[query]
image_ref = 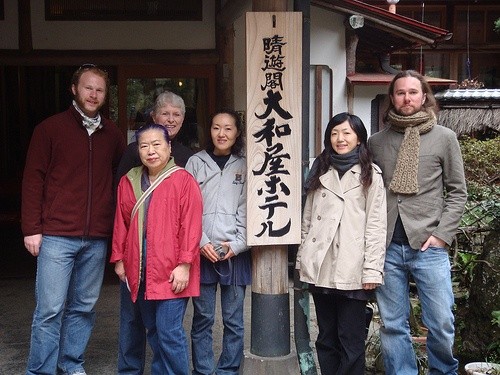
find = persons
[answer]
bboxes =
[365,70,468,375]
[109,123,203,375]
[21,63,120,374]
[112,92,193,375]
[186,110,255,374]
[295,114,387,375]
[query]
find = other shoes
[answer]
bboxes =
[67,371,87,375]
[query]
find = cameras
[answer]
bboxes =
[213,244,229,259]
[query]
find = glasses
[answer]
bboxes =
[74,63,95,74]
